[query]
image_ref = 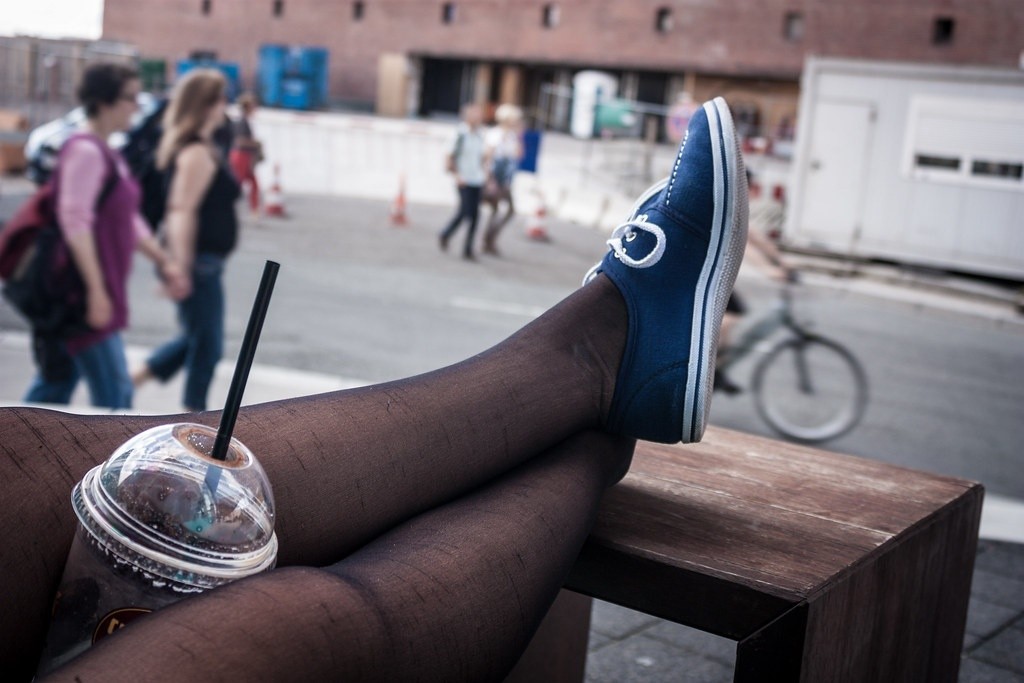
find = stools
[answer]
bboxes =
[505,425,988,681]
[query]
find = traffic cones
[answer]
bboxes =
[260,163,288,220]
[388,183,409,230]
[522,195,551,243]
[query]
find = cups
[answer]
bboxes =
[27,471,278,683]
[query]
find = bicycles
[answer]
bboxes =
[710,260,873,446]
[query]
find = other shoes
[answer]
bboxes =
[437,234,447,248]
[462,250,477,262]
[480,240,499,257]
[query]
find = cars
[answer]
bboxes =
[21,91,161,191]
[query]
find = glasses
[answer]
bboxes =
[121,93,139,103]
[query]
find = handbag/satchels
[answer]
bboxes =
[0,133,119,342]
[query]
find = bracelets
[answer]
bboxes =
[154,251,170,271]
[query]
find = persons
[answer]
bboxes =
[21,59,187,410]
[230,94,262,213]
[130,69,242,413]
[124,99,171,167]
[438,102,541,261]
[715,165,801,394]
[0,94,752,683]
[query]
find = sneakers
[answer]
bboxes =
[580,96,749,444]
